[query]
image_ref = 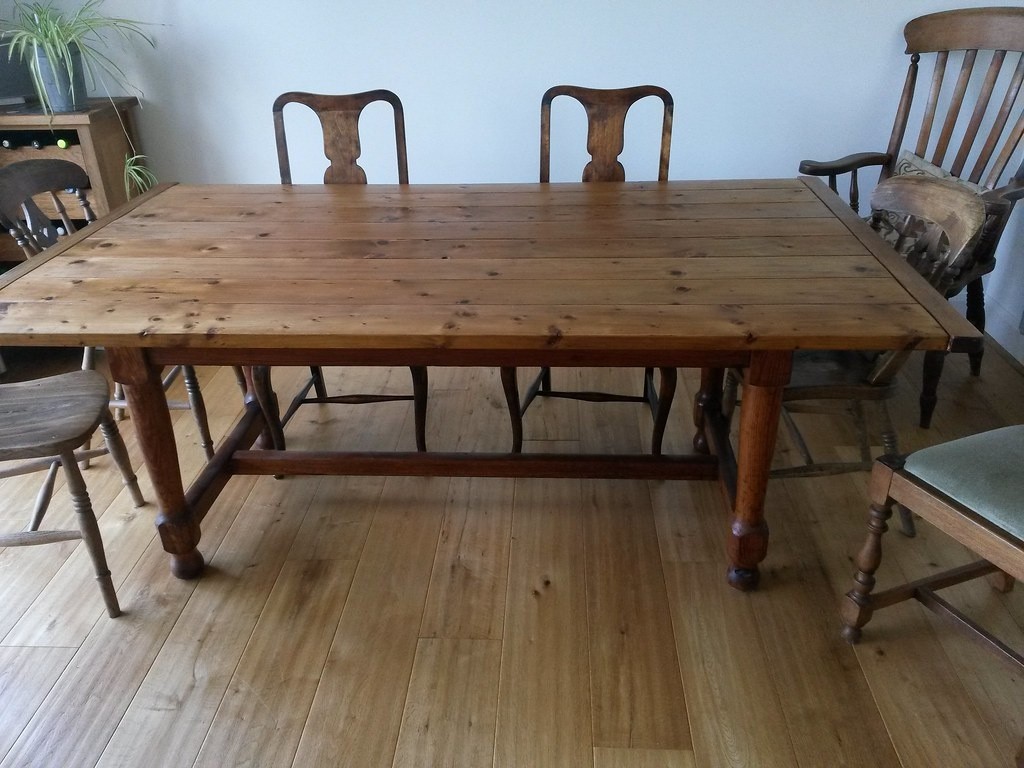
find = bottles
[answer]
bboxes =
[55,226,68,236]
[1,131,79,149]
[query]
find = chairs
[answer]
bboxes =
[796,7,1024,429]
[496,83,681,454]
[0,369,150,619]
[0,158,248,470]
[721,174,989,541]
[246,87,432,481]
[838,419,1024,764]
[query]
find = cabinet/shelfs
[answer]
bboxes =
[0,97,140,360]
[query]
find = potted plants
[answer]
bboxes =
[0,0,173,204]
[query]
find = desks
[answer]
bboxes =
[0,177,987,590]
